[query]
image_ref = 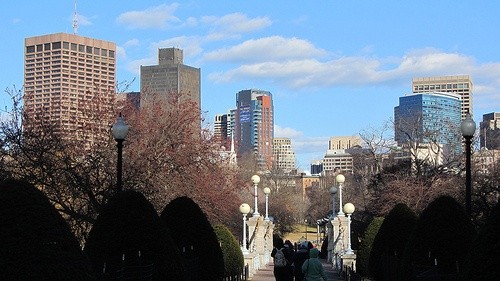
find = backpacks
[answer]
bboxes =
[275,248,289,266]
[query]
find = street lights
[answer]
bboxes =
[329,185,338,218]
[251,175,261,218]
[263,186,271,221]
[239,203,250,252]
[343,202,355,254]
[460,112,476,281]
[335,174,347,217]
[110,111,130,197]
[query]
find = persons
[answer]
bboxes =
[284,239,328,281]
[271,238,294,281]
